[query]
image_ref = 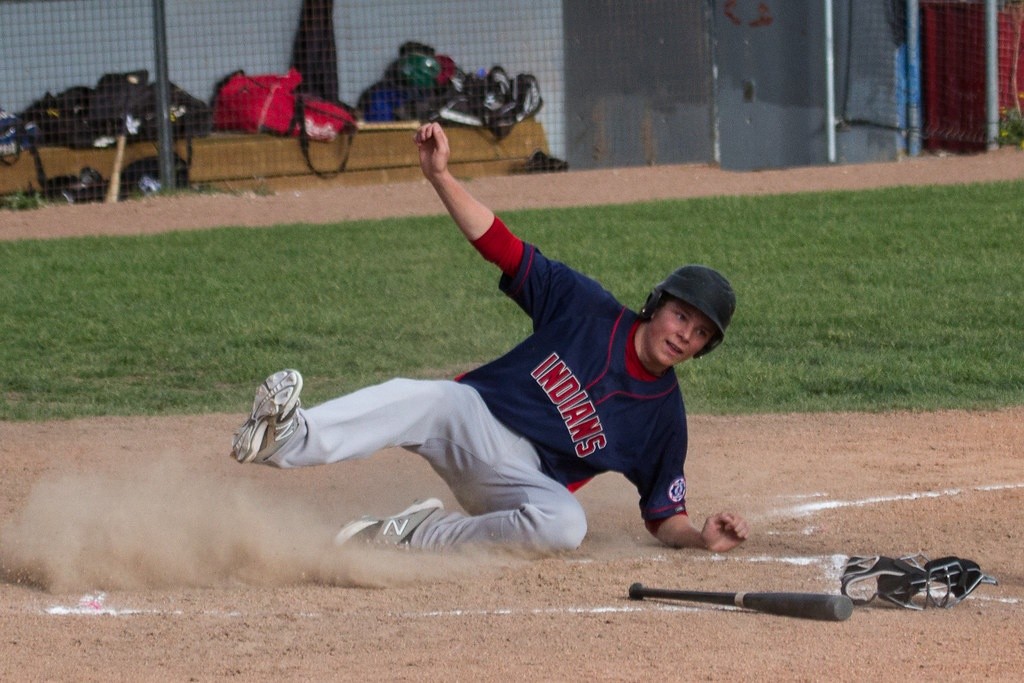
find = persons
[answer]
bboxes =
[231,122,750,558]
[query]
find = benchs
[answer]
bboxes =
[0,116,548,195]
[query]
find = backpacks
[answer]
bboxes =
[360,42,544,136]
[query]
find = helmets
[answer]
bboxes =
[646,266,736,358]
[394,55,445,89]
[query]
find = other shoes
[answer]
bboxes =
[512,151,569,173]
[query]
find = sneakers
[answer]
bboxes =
[332,499,445,549]
[231,367,303,463]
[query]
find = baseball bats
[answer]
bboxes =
[627,580,856,624]
[103,74,139,204]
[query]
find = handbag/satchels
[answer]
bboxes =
[210,71,357,180]
[0,69,208,203]
